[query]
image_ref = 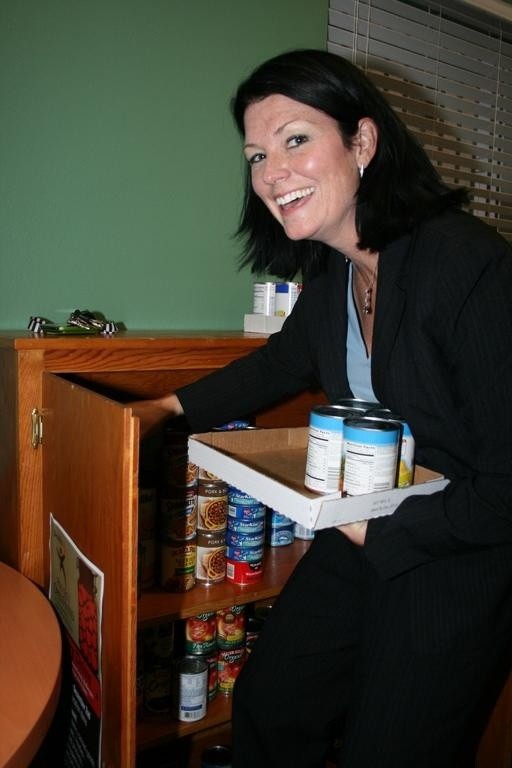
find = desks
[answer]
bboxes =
[0,561,64,768]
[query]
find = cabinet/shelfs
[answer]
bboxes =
[0,330,338,767]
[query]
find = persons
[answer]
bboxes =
[127,51,511,766]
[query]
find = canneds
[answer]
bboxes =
[253,281,303,318]
[136,604,272,723]
[139,418,317,593]
[301,399,416,497]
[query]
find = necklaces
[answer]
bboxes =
[354,260,380,316]
